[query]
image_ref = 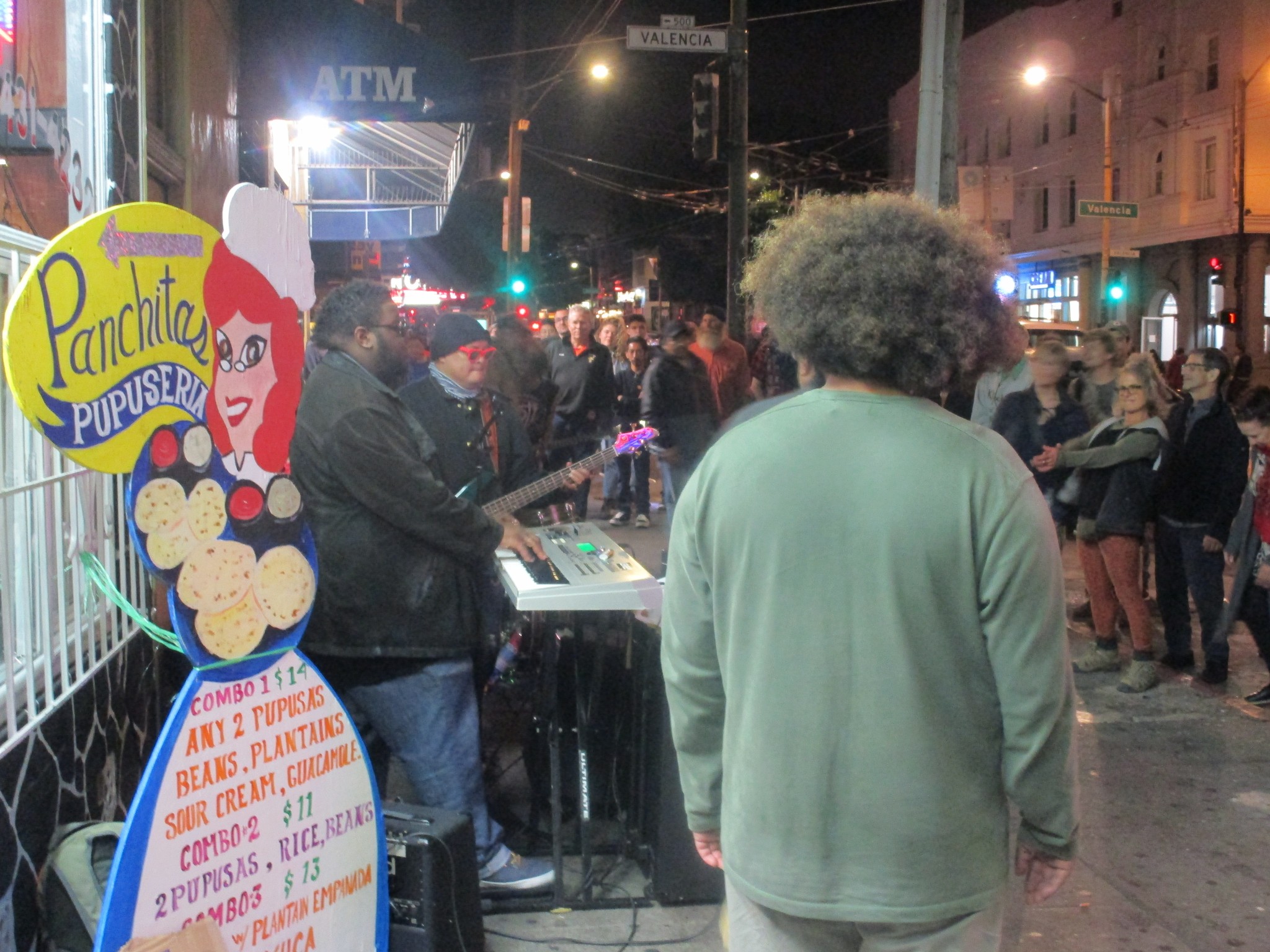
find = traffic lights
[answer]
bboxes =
[1209,257,1224,287]
[1106,283,1126,304]
[1221,309,1237,328]
[513,279,526,296]
[505,64,611,308]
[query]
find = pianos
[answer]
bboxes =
[489,521,665,913]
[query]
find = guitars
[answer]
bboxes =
[483,419,659,522]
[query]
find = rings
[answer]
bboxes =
[1043,459,1045,466]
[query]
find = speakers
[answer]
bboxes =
[634,622,724,904]
[380,800,487,952]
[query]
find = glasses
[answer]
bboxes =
[1181,361,1202,368]
[373,316,407,336]
[459,345,495,362]
[1115,384,1140,394]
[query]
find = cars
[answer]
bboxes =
[1018,318,1087,358]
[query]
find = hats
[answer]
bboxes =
[662,320,687,337]
[702,307,726,322]
[429,313,491,360]
[1105,319,1129,338]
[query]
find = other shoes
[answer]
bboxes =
[1073,638,1120,673]
[609,511,630,525]
[1117,650,1157,692]
[1161,649,1193,667]
[635,515,650,528]
[1067,601,1094,635]
[1194,661,1227,684]
[1244,683,1270,705]
[481,847,555,889]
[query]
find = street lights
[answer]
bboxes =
[1019,57,1117,328]
[570,260,595,313]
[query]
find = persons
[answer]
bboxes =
[660,185,1086,952]
[303,298,1270,852]
[285,278,561,888]
